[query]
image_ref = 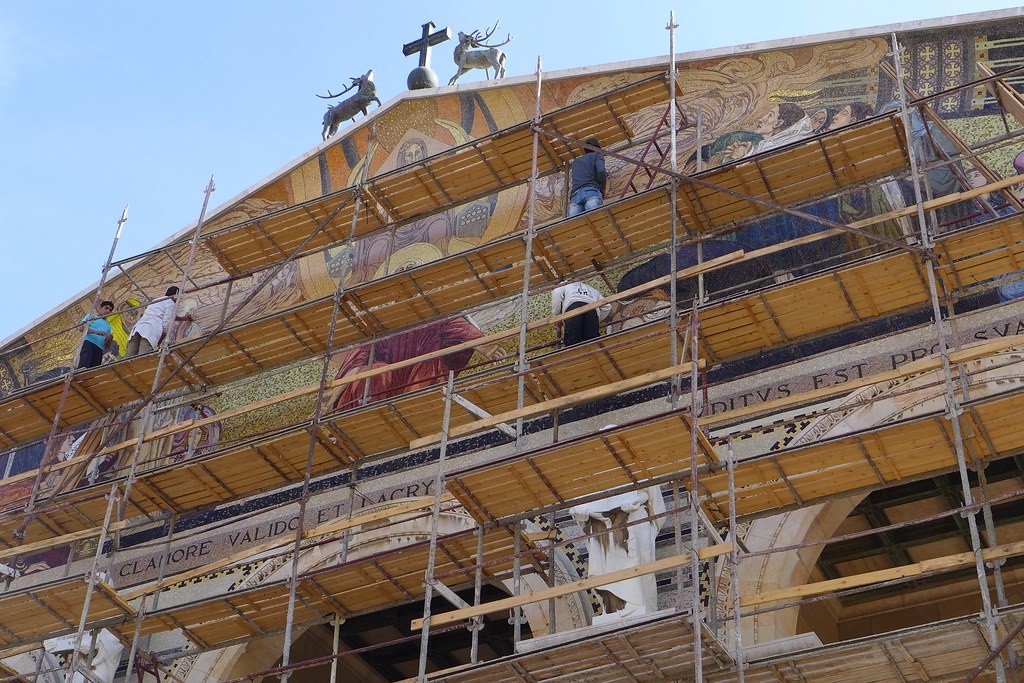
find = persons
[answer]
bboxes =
[567,138,607,219]
[551,282,611,347]
[126,285,193,358]
[78,301,114,369]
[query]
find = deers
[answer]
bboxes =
[315,69,381,141]
[448,20,515,86]
